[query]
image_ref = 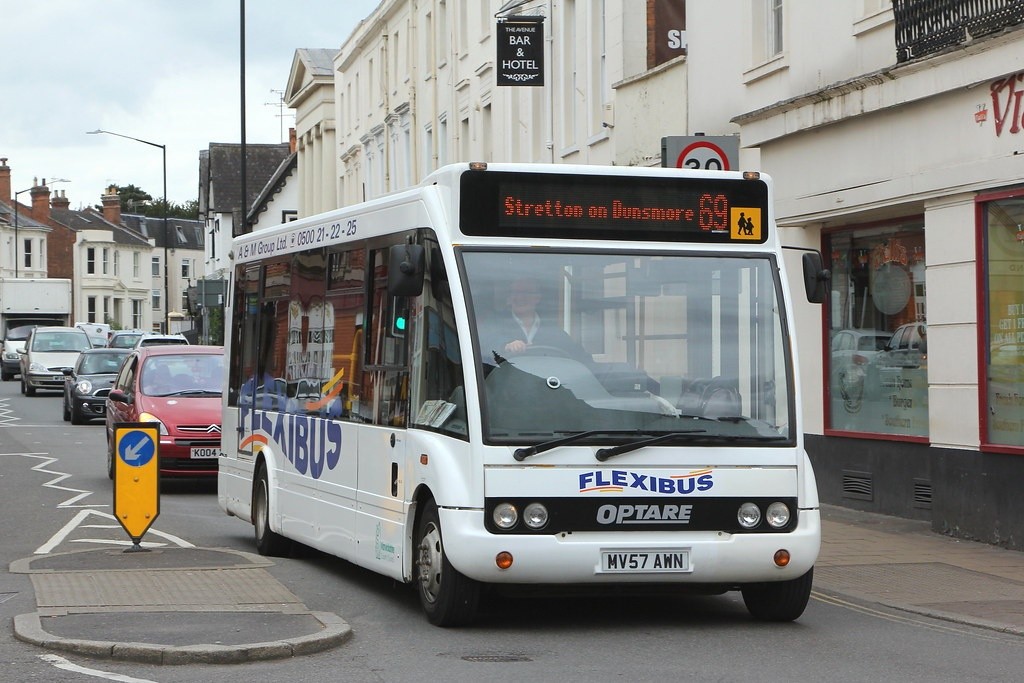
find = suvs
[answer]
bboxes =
[832,330,894,399]
[865,323,927,402]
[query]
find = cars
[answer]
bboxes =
[17,325,95,397]
[237,377,358,416]
[104,346,224,480]
[61,348,137,424]
[988,339,1024,416]
[107,330,156,360]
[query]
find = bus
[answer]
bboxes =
[218,161,832,622]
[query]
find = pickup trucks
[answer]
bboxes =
[132,332,199,377]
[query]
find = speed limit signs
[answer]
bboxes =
[677,145,730,172]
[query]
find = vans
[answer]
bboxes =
[73,322,112,348]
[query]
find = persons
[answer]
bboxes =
[143,364,178,395]
[483,274,594,371]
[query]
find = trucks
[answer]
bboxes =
[0,277,73,383]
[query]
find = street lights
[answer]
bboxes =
[86,129,168,336]
[14,178,72,277]
[197,304,209,345]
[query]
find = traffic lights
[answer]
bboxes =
[387,292,407,339]
